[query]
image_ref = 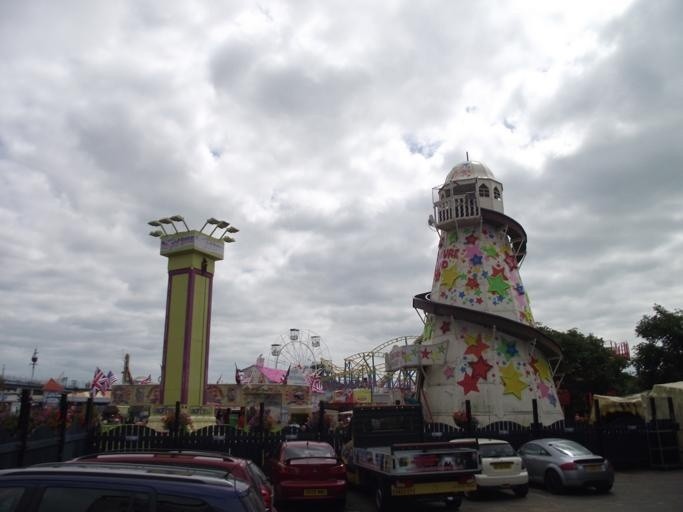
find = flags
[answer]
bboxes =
[90,367,118,397]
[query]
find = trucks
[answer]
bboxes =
[341,399,482,511]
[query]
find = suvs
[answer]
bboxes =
[0,461,265,512]
[65,449,278,512]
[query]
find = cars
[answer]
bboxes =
[276,441,347,501]
[452,424,615,500]
